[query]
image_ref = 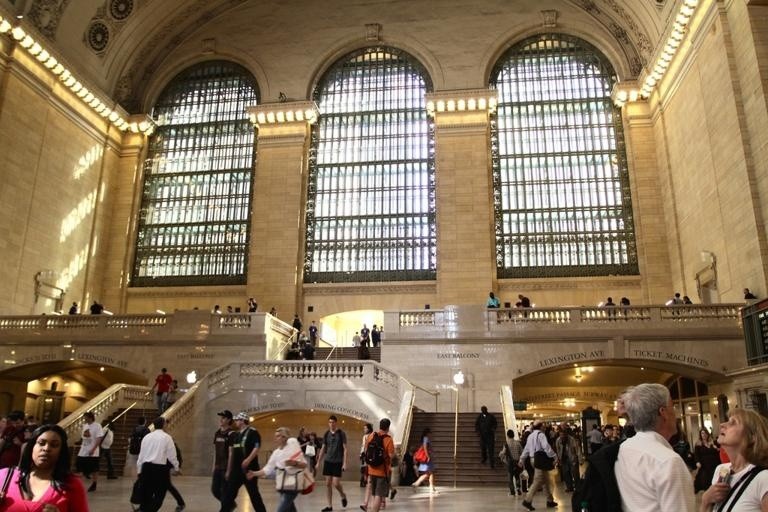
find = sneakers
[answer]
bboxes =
[321,488,397,512]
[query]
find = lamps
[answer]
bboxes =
[1,12,159,139]
[610,0,701,110]
[247,107,321,127]
[421,94,498,117]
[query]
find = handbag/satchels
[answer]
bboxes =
[302,467,316,494]
[506,447,524,475]
[274,465,304,492]
[534,449,555,470]
[415,448,427,463]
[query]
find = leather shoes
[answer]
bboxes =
[522,500,535,511]
[546,501,558,508]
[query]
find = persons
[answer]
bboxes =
[220,412,269,512]
[671,292,682,315]
[604,296,617,321]
[1,409,113,512]
[353,323,381,360]
[225,306,232,313]
[165,381,181,402]
[246,297,257,311]
[683,295,692,304]
[486,292,501,310]
[164,445,185,512]
[209,410,237,512]
[213,303,222,313]
[133,417,152,462]
[298,412,399,511]
[411,427,437,493]
[620,296,631,320]
[742,287,756,300]
[130,415,182,512]
[89,300,102,314]
[515,302,522,308]
[285,314,317,361]
[269,307,276,317]
[149,368,173,414]
[500,384,768,512]
[516,294,529,320]
[247,427,308,512]
[475,404,497,469]
[69,301,81,314]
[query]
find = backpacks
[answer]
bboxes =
[129,426,146,454]
[365,432,391,467]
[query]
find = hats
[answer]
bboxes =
[233,412,249,420]
[217,410,233,419]
